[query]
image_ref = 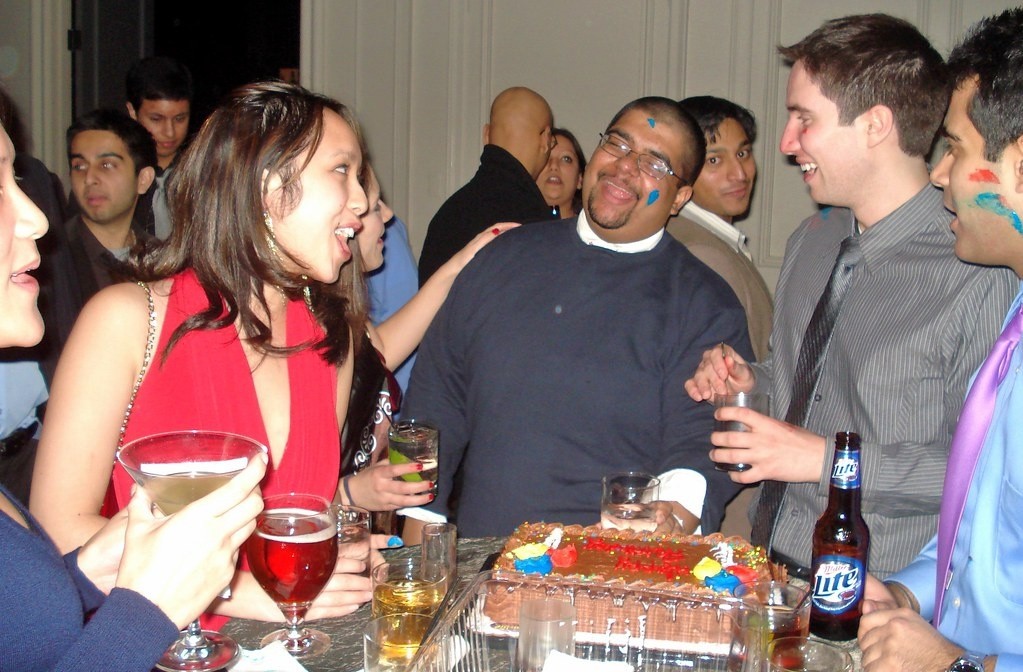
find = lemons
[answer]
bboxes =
[388,448,422,482]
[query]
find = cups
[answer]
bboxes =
[763,637,852,672]
[325,505,372,608]
[729,581,813,672]
[371,558,448,646]
[420,522,457,588]
[363,613,437,672]
[713,392,769,474]
[516,599,577,672]
[601,470,661,534]
[388,421,438,498]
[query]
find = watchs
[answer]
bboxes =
[945,650,987,672]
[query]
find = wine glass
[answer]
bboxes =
[116,430,267,672]
[246,493,338,660]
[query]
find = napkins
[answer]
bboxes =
[226,640,308,672]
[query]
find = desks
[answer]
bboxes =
[218,536,858,672]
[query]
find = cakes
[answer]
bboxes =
[483,521,789,645]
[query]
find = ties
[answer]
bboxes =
[932,305,1023,630]
[750,236,862,557]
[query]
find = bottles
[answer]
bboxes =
[548,204,561,220]
[808,432,871,641]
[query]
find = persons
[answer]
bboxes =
[534,127,587,219]
[685,12,1020,592]
[397,94,756,544]
[798,3,1023,672]
[416,88,563,289]
[0,52,519,672]
[665,95,776,365]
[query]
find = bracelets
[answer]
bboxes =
[343,474,357,507]
[888,581,915,611]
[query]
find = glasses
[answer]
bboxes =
[599,133,690,186]
